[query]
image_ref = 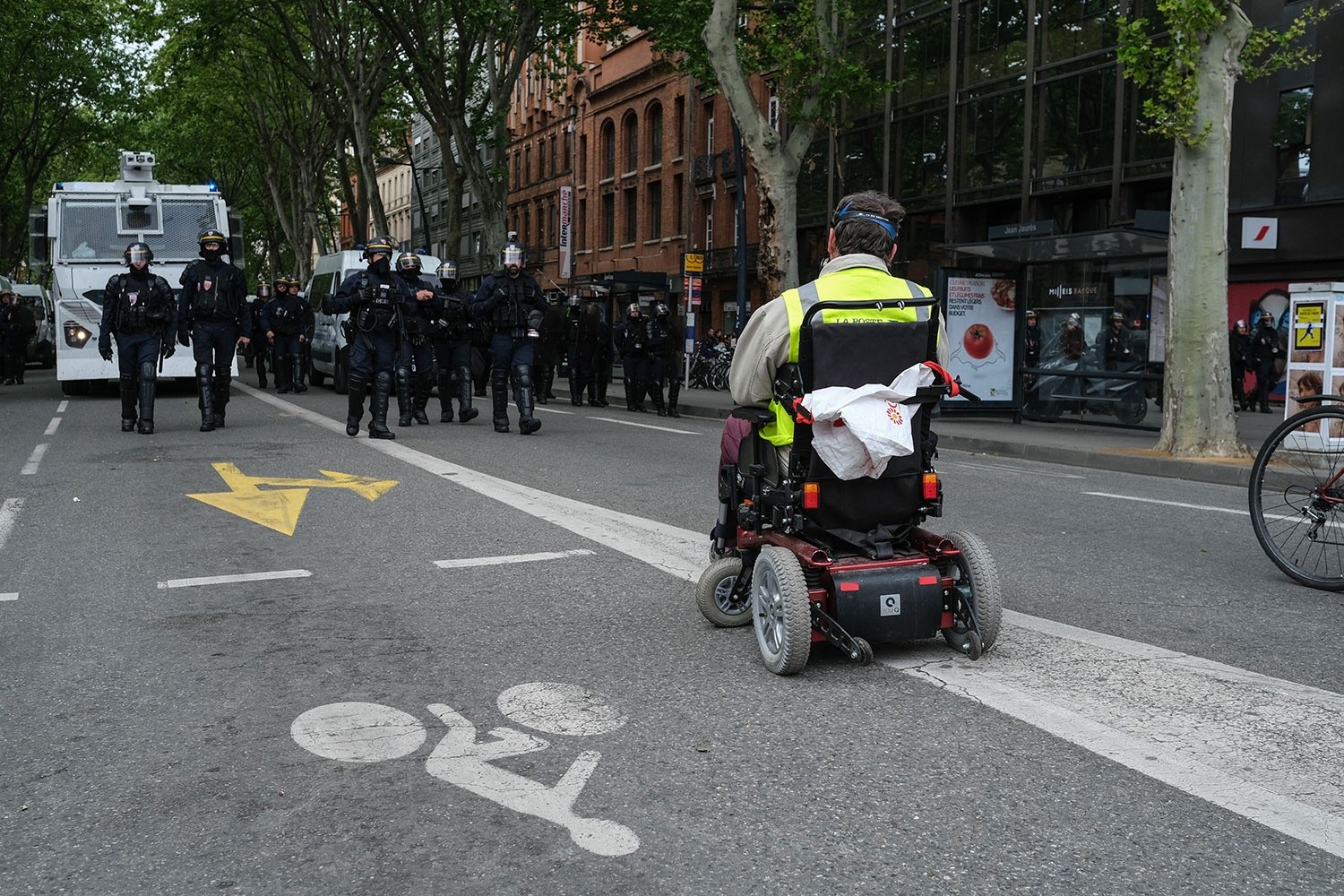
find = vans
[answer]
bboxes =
[0,274,57,371]
[302,244,442,397]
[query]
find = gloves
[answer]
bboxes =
[177,330,190,347]
[98,343,114,361]
[525,287,537,300]
[360,287,372,301]
[495,284,509,298]
[162,341,176,358]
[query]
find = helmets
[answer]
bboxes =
[569,293,582,306]
[395,251,422,274]
[626,303,642,316]
[1234,319,1248,328]
[257,281,270,296]
[361,236,392,261]
[11,293,24,306]
[273,274,302,288]
[1,288,12,296]
[1111,312,1124,320]
[654,303,672,316]
[124,242,154,268]
[500,241,526,266]
[1260,312,1274,318]
[197,227,225,245]
[435,260,460,282]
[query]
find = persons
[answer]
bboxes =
[1023,310,1041,367]
[1244,312,1288,414]
[0,289,37,385]
[98,242,177,435]
[1094,312,1131,372]
[329,237,413,440]
[473,289,739,418]
[1228,319,1254,411]
[253,274,315,394]
[1058,313,1090,358]
[473,240,547,435]
[393,252,444,427]
[717,189,951,499]
[177,228,251,432]
[1292,372,1322,433]
[434,261,479,423]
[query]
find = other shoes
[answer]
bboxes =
[458,407,479,423]
[398,415,412,427]
[259,378,308,394]
[411,408,429,425]
[441,409,454,422]
[136,418,154,435]
[1246,395,1256,412]
[492,416,510,433]
[1260,403,1273,414]
[121,418,136,432]
[518,418,542,435]
[657,406,666,417]
[346,414,363,436]
[1238,394,1247,411]
[200,414,226,432]
[367,420,396,440]
[572,394,648,412]
[5,377,24,386]
[667,408,680,418]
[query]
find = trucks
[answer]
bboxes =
[45,150,247,396]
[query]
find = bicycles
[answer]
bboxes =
[1247,393,1344,595]
[679,339,733,394]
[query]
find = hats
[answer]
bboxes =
[1026,311,1036,317]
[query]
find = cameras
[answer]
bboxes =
[508,231,518,240]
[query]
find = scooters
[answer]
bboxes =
[695,294,1005,677]
[1021,326,1153,424]
